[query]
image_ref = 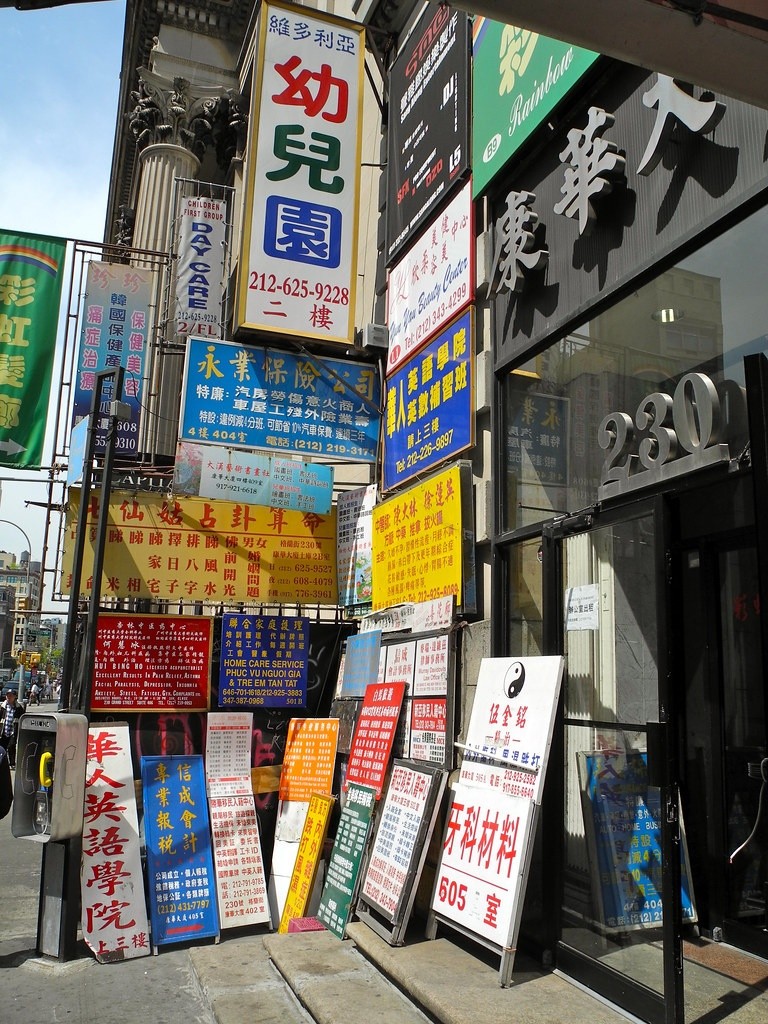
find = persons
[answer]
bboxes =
[0,705,13,820]
[56,683,62,709]
[0,689,25,770]
[28,681,53,706]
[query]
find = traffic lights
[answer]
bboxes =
[19,652,26,663]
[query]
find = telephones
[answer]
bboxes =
[39,751,53,789]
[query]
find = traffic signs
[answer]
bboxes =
[14,633,36,643]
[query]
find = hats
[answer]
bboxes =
[6,689,16,696]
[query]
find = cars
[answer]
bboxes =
[1,680,20,701]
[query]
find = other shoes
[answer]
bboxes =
[10,765,16,770]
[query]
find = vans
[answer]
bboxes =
[0,668,52,699]
